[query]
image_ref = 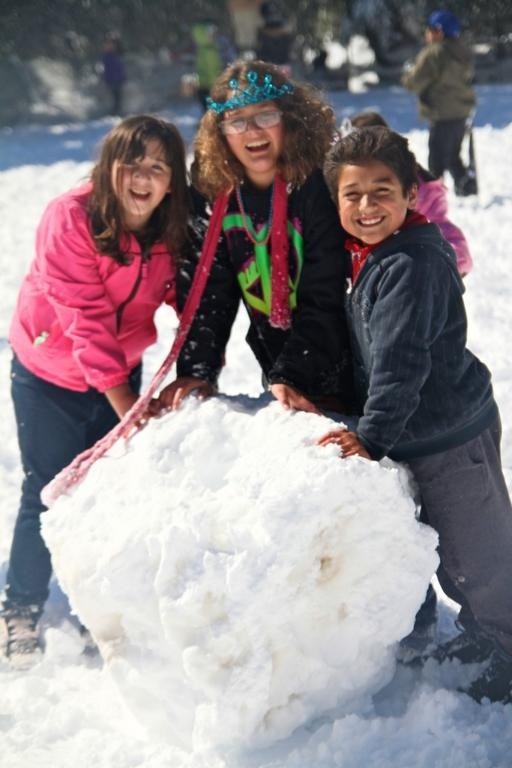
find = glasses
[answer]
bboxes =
[218,110,284,135]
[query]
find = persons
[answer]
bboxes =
[95,30,126,119]
[312,122,511,710]
[2,110,193,669]
[186,26,222,112]
[336,111,476,282]
[400,9,481,197]
[158,52,440,664]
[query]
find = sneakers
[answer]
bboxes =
[399,584,438,668]
[412,618,495,671]
[1,604,45,668]
[457,660,512,706]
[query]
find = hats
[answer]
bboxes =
[423,10,461,38]
